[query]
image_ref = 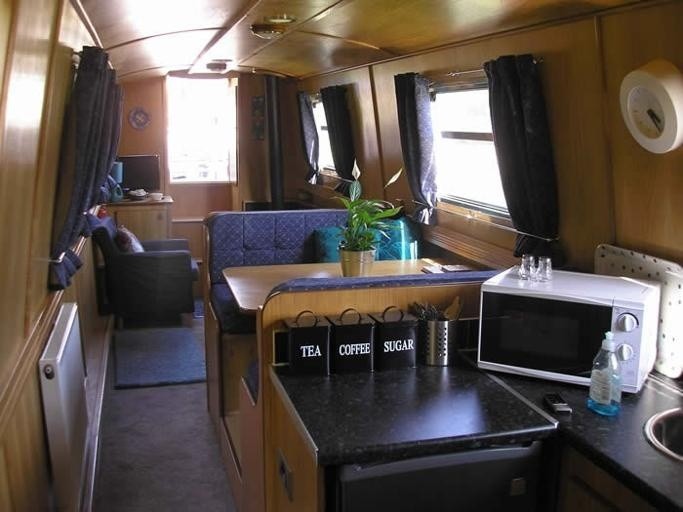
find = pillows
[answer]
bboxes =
[374,214,432,259]
[311,226,381,263]
[115,222,145,253]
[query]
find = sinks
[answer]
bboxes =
[644,407,683,461]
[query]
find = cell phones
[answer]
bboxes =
[542,392,573,414]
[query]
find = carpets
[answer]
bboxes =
[189,293,205,318]
[113,327,206,388]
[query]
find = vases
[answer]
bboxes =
[337,245,377,277]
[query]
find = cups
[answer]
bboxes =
[519,254,535,280]
[536,257,553,282]
[149,193,162,201]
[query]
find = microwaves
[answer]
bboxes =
[477,264,662,393]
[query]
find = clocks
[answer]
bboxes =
[618,58,682,155]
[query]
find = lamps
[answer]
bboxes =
[112,162,123,184]
[250,22,292,42]
[206,63,227,73]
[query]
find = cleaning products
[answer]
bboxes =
[587,331,623,416]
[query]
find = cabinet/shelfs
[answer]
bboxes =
[103,194,175,241]
[556,442,654,512]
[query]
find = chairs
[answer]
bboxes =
[93,216,200,314]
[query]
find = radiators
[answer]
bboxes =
[38,300,90,512]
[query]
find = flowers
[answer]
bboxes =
[329,158,403,252]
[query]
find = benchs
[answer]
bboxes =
[199,210,423,421]
[242,269,512,512]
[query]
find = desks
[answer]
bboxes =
[222,258,471,311]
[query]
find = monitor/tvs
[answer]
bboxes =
[116,155,160,191]
